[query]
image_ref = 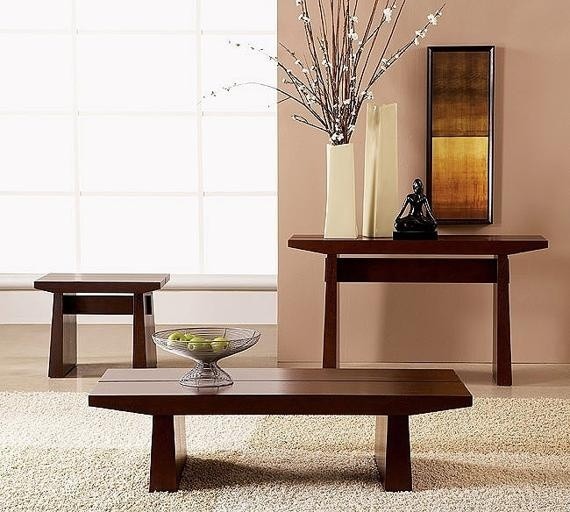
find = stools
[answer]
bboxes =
[33,272,170,378]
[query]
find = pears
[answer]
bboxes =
[166,332,232,354]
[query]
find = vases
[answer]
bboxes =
[361,100,399,238]
[324,143,358,239]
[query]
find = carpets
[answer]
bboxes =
[0,390,570,511]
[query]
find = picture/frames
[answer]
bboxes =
[427,44,495,226]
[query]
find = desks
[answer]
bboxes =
[88,368,473,492]
[286,233,550,386]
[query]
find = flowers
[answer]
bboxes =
[199,0,448,146]
